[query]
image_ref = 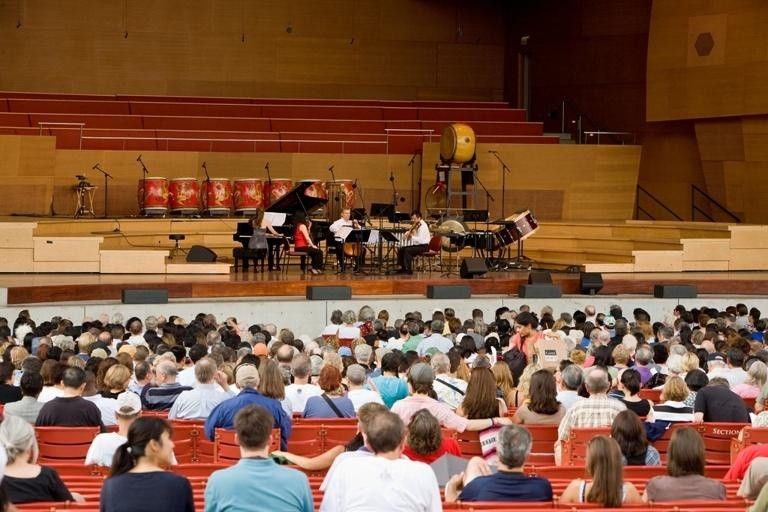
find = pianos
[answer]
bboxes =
[233,183,330,271]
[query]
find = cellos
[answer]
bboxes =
[344,210,364,258]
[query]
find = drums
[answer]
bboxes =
[497,208,539,247]
[439,124,475,165]
[137,176,355,215]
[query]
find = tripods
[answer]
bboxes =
[94,175,120,219]
[440,236,461,278]
[127,170,165,218]
[194,182,222,218]
[334,213,401,276]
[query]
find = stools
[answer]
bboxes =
[168,234,185,260]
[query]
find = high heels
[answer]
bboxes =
[311,269,324,275]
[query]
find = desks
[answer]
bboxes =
[71,186,99,217]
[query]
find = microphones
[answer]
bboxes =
[265,162,269,169]
[136,155,141,161]
[329,165,334,171]
[92,163,99,169]
[202,161,205,168]
[488,151,497,153]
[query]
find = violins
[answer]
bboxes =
[407,222,422,240]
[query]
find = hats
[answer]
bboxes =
[604,316,616,326]
[410,363,435,383]
[236,365,259,388]
[116,391,141,416]
[338,346,353,356]
[707,353,727,363]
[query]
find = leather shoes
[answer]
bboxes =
[396,270,413,275]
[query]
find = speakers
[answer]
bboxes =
[461,258,488,278]
[579,272,603,295]
[186,245,217,262]
[528,272,553,285]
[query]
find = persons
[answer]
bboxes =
[246,204,284,271]
[203,404,315,511]
[721,441,767,512]
[445,423,554,503]
[99,415,196,511]
[642,426,727,500]
[327,207,365,272]
[1,301,766,471]
[0,415,76,502]
[292,210,325,276]
[392,209,432,275]
[557,434,643,504]
[321,411,442,511]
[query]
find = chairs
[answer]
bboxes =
[282,235,443,274]
[0,335,768,512]
